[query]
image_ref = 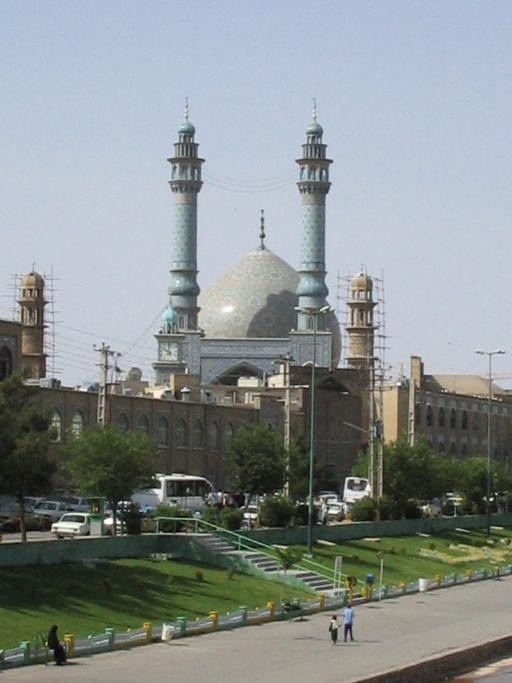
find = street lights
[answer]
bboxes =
[293,306,334,554]
[476,349,505,536]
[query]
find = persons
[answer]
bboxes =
[321,499,329,524]
[329,615,339,643]
[186,486,245,509]
[344,604,353,642]
[48,625,66,666]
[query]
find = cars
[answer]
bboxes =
[239,505,260,527]
[1,495,132,537]
[315,489,345,521]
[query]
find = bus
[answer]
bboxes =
[134,473,217,515]
[342,476,372,519]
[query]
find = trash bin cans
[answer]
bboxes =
[140,512,154,533]
[161,624,174,641]
[418,578,430,592]
